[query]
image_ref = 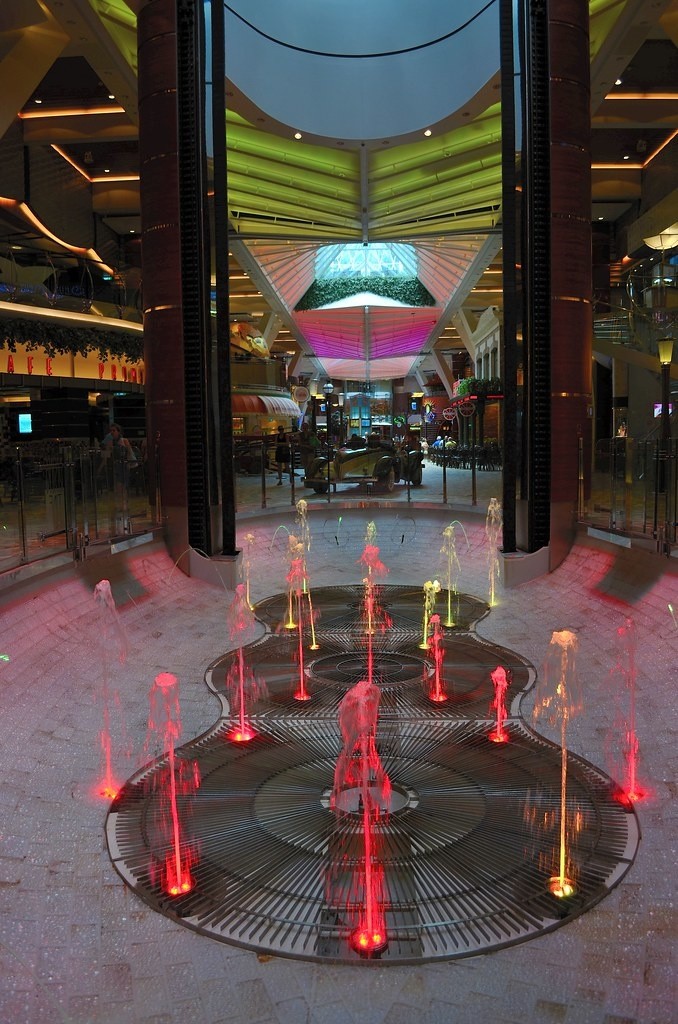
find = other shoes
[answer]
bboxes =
[277,482,282,485]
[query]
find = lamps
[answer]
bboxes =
[657,335,676,363]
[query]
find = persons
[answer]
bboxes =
[107,424,138,473]
[421,436,456,454]
[274,423,328,486]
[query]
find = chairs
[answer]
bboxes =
[428,446,501,471]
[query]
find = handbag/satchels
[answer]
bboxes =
[96,453,114,483]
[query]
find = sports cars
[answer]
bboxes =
[303,441,427,495]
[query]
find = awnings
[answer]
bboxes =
[232,394,301,417]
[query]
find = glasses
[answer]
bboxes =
[279,429,284,430]
